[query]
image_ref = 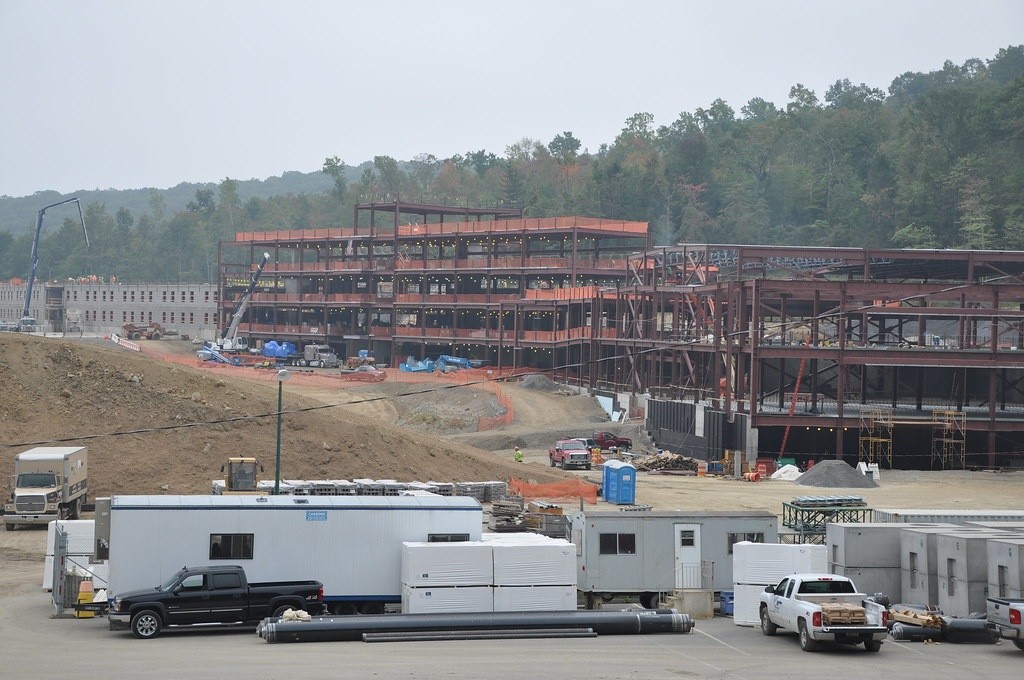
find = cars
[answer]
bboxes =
[1,321,21,333]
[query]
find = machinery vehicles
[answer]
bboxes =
[18,195,91,332]
[217,453,266,496]
[212,253,270,352]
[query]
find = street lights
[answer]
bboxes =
[273,363,288,493]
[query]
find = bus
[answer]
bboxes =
[122,321,163,341]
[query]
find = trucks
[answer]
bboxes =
[4,444,87,530]
[285,345,338,369]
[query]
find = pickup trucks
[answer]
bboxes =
[338,365,386,383]
[586,432,632,454]
[759,575,889,656]
[984,596,1023,651]
[111,563,325,639]
[548,437,591,471]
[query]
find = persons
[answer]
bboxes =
[34,274,117,285]
[514,446,523,462]
